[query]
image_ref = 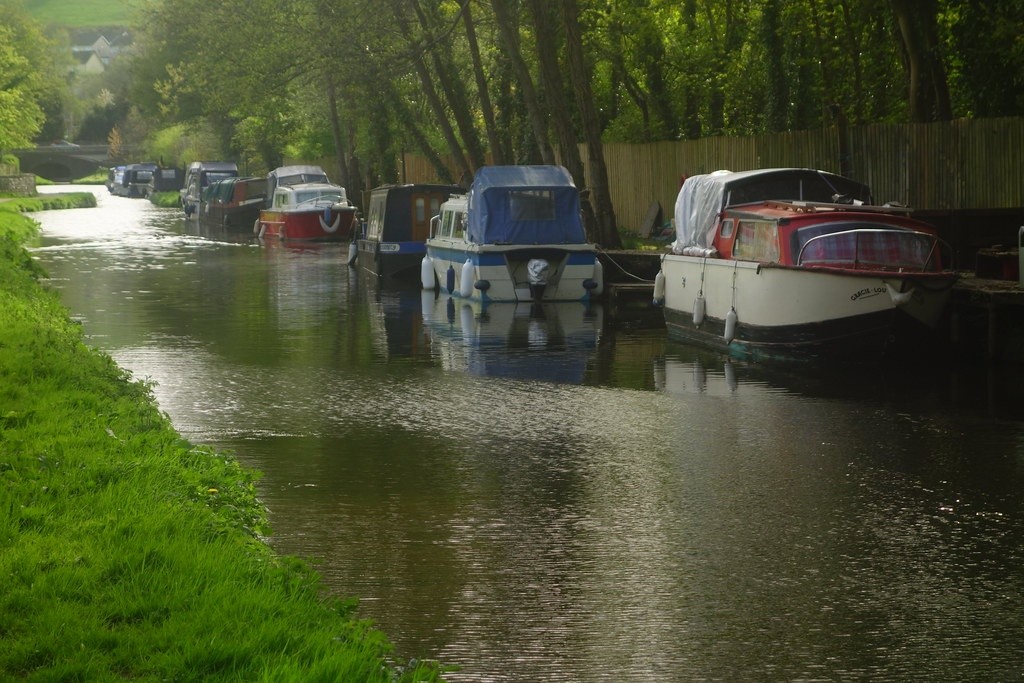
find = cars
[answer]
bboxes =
[49,140,80,152]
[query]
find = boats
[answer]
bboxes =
[653,167,959,376]
[108,162,186,200]
[347,184,467,279]
[420,288,605,359]
[420,165,604,303]
[253,183,358,242]
[266,164,332,208]
[180,162,266,225]
